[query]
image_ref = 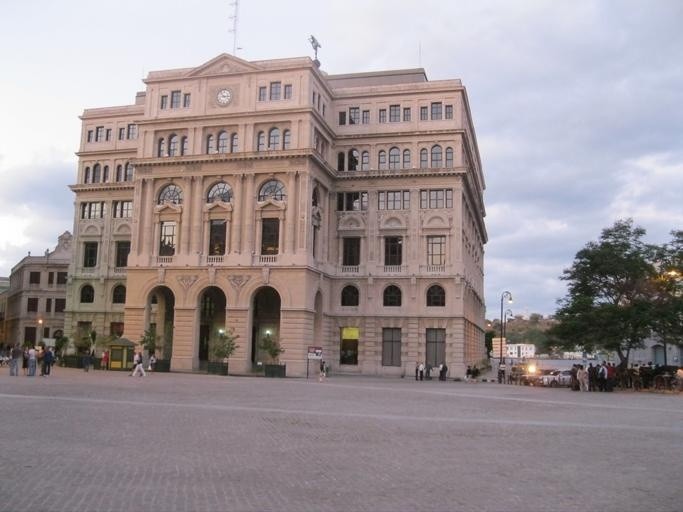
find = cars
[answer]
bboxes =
[511,365,572,388]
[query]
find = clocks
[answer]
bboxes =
[214,87,232,105]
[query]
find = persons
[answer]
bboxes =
[0,342,157,377]
[319,359,327,377]
[414,357,679,392]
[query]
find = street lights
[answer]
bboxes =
[658,258,678,373]
[497,291,513,383]
[504,309,513,337]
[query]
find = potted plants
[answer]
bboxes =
[140,324,170,372]
[55,325,103,369]
[205,326,239,376]
[258,333,288,379]
